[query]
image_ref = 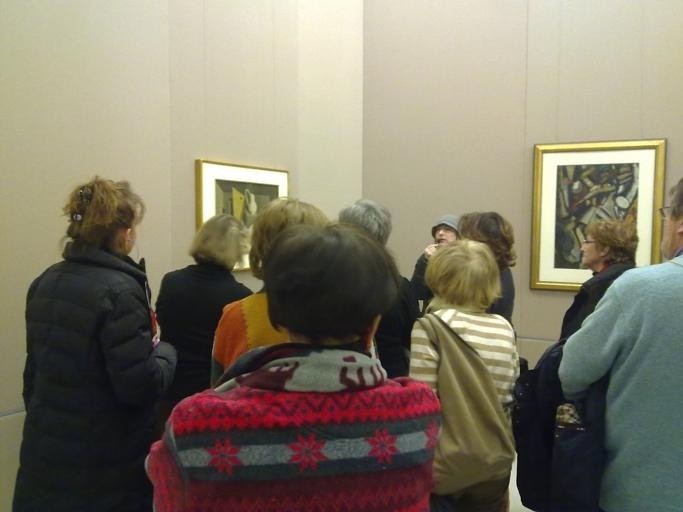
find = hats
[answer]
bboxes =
[432,214,459,236]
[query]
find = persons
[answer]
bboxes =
[154,195,522,511]
[559,213,638,340]
[12,175,176,512]
[556,177,683,512]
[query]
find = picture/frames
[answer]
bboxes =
[195,158,289,272]
[530,137,666,290]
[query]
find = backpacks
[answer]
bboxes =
[512,338,577,511]
